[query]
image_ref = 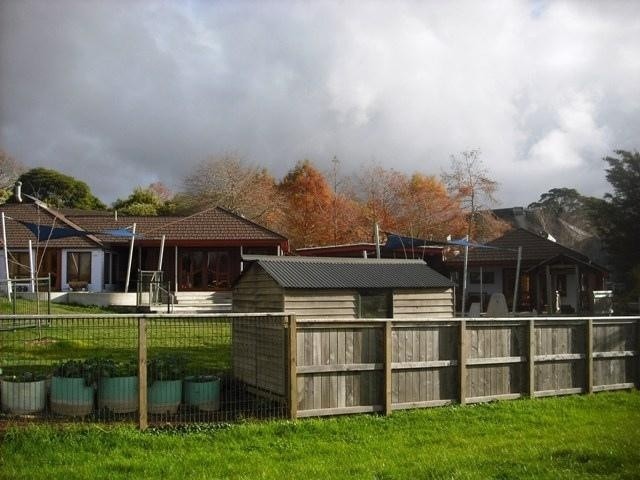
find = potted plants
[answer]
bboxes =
[95,354,139,414]
[50,360,95,416]
[145,360,182,414]
[183,376,221,412]
[1,370,47,416]
